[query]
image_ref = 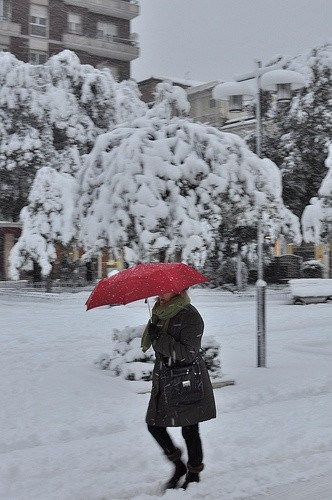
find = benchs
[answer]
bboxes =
[287,279,332,306]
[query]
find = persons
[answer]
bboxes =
[140,287,217,489]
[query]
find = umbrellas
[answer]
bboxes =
[84,262,208,324]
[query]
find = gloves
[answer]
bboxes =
[147,322,160,340]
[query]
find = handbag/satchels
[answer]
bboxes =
[163,363,204,407]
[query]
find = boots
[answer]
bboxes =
[165,450,187,488]
[180,463,205,489]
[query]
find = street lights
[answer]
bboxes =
[212,70,309,369]
